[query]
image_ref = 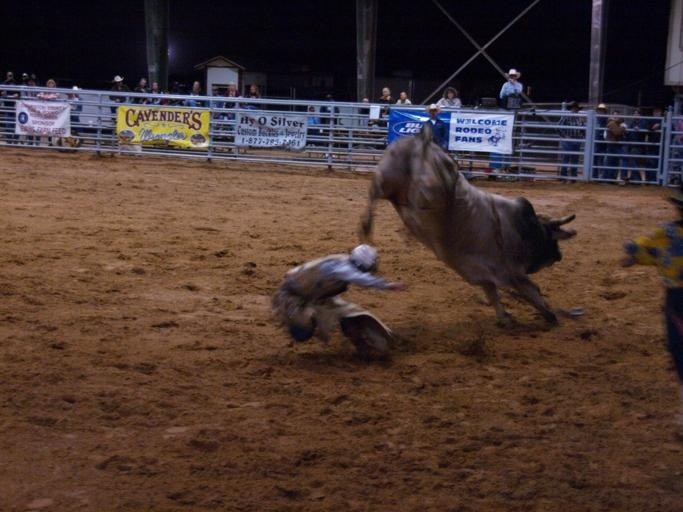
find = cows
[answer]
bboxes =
[359,122,576,332]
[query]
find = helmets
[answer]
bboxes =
[350,243,378,271]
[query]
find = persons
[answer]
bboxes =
[556,101,672,183]
[271,241,408,368]
[2,72,85,147]
[306,93,339,147]
[617,188,683,387]
[364,69,523,177]
[114,75,261,108]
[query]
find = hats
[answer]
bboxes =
[426,103,440,114]
[503,68,521,80]
[444,86,458,98]
[566,100,583,110]
[596,102,608,112]
[111,76,125,83]
